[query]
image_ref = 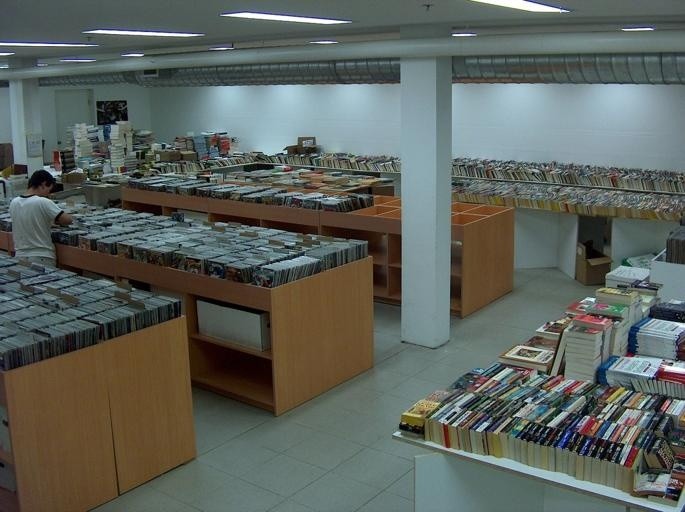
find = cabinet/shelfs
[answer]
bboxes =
[0,201,375,512]
[121,184,514,320]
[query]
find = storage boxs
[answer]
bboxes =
[577,240,611,285]
